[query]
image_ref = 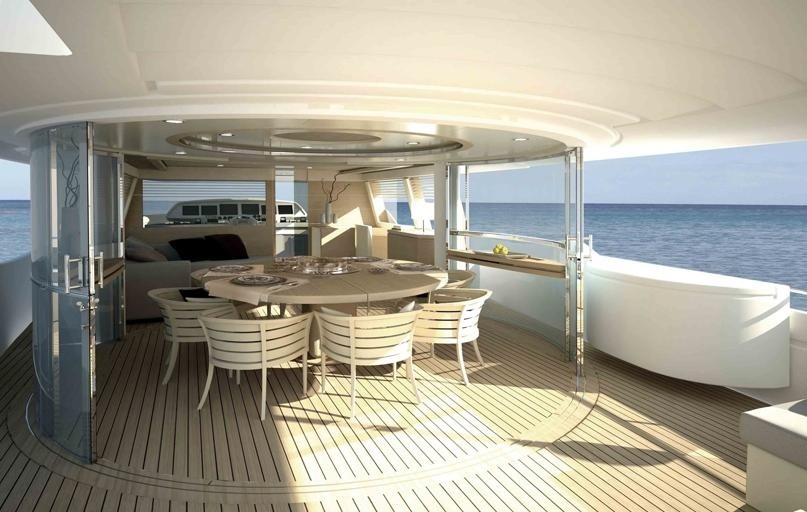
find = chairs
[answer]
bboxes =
[446,268,478,288]
[197,304,314,422]
[149,286,240,386]
[305,301,424,420]
[406,287,493,386]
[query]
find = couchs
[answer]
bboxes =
[125,238,272,322]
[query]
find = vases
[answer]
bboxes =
[325,202,332,224]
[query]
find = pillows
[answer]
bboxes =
[125,233,249,262]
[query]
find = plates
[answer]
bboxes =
[341,256,438,271]
[208,264,289,286]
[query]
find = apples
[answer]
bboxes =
[493,244,508,254]
